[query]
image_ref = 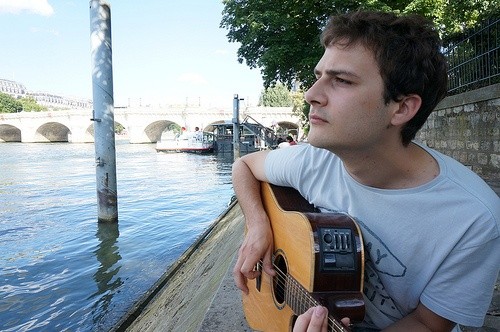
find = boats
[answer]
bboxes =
[155,125,217,154]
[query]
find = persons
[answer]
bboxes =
[278,136,297,149]
[231,6,499,332]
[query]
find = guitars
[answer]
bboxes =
[240,181,368,332]
